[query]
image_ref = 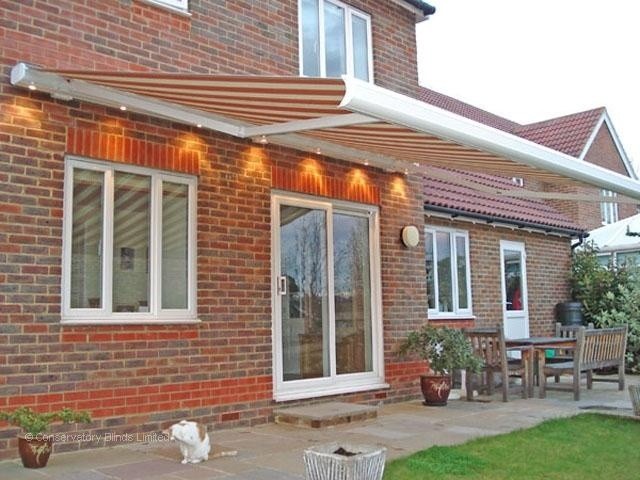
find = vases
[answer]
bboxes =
[301,439,388,480]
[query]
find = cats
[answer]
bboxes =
[162,420,238,464]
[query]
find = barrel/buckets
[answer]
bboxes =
[556,302,585,337]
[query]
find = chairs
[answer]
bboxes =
[461,324,530,402]
[556,320,595,383]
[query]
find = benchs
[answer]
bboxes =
[539,323,629,401]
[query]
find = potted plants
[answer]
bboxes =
[0,405,94,468]
[392,322,484,406]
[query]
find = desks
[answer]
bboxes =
[482,337,577,398]
